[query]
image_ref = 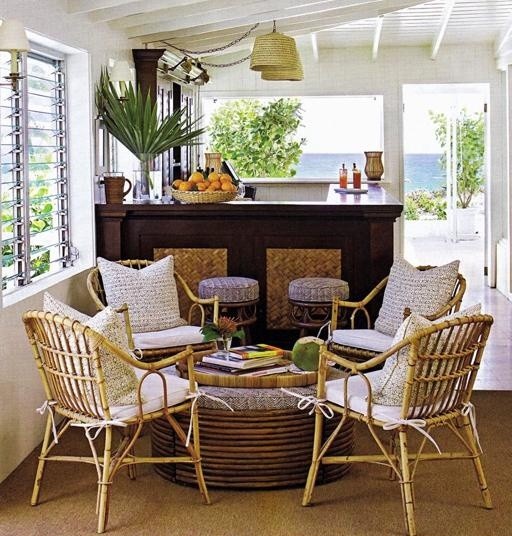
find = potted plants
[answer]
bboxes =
[93,63,208,205]
[426,105,488,244]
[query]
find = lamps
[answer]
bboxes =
[246,18,304,87]
[1,17,32,92]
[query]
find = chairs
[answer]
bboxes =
[327,264,467,437]
[22,309,212,536]
[85,259,220,364]
[301,314,494,535]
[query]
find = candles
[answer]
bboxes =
[351,162,361,189]
[339,163,348,188]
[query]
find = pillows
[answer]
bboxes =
[43,291,148,407]
[97,255,188,332]
[366,303,481,407]
[374,253,460,339]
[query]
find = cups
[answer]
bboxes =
[353,170,361,189]
[364,151,384,181]
[205,152,222,172]
[103,176,132,204]
[339,168,348,188]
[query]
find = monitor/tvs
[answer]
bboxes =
[222,160,240,181]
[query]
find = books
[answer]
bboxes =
[192,341,291,377]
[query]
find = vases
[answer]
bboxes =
[204,152,223,174]
[361,151,385,182]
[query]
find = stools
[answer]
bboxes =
[286,276,349,346]
[198,276,261,347]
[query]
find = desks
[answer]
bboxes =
[146,365,354,490]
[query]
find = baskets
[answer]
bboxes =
[170,189,237,206]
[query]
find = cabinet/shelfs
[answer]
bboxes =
[129,43,211,197]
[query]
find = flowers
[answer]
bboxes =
[201,315,243,351]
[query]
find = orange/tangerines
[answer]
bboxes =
[172,171,238,192]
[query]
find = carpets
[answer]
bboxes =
[1,390,511,536]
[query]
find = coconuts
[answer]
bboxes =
[291,336,326,371]
